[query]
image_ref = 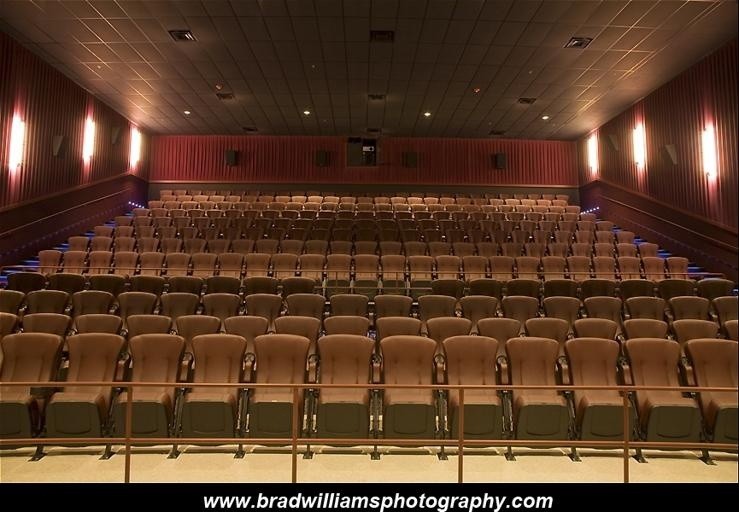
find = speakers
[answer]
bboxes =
[52,135,69,158]
[315,149,327,167]
[225,149,237,166]
[404,151,418,168]
[110,126,123,145]
[496,152,507,169]
[605,134,619,152]
[659,143,678,166]
